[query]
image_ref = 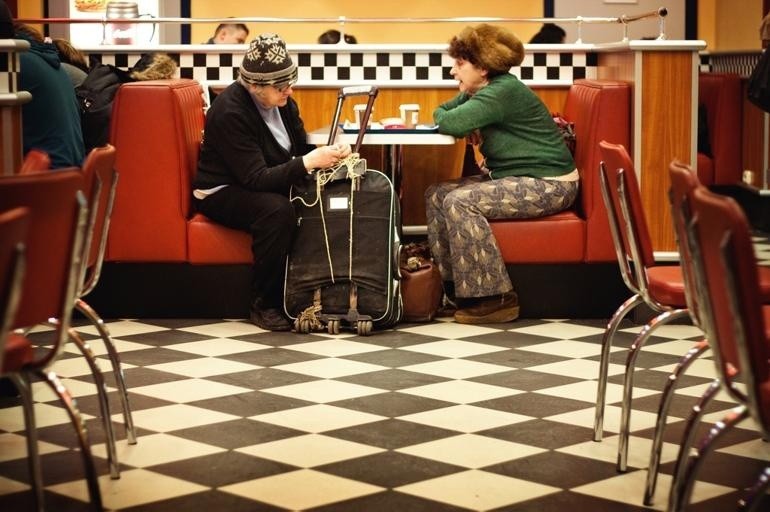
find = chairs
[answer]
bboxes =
[595,141,692,472]
[1,206,47,511]
[23,141,137,483]
[675,182,762,505]
[3,171,112,512]
[646,159,763,505]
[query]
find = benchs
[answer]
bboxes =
[108,78,254,314]
[471,78,629,320]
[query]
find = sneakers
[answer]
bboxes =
[455,292,524,324]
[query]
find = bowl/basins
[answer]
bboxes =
[379,116,403,125]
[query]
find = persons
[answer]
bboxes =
[203,17,248,46]
[192,34,352,331]
[528,23,566,44]
[0,0,86,171]
[11,20,89,93]
[424,25,581,325]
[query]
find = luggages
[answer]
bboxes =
[283,84,404,335]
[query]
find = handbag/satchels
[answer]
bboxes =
[746,46,769,112]
[399,256,443,322]
[74,64,128,151]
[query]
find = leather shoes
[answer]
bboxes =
[247,303,290,331]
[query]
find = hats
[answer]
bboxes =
[241,34,299,86]
[463,22,526,72]
[127,53,178,80]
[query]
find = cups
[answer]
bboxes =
[353,103,375,130]
[398,103,420,130]
[102,2,156,46]
